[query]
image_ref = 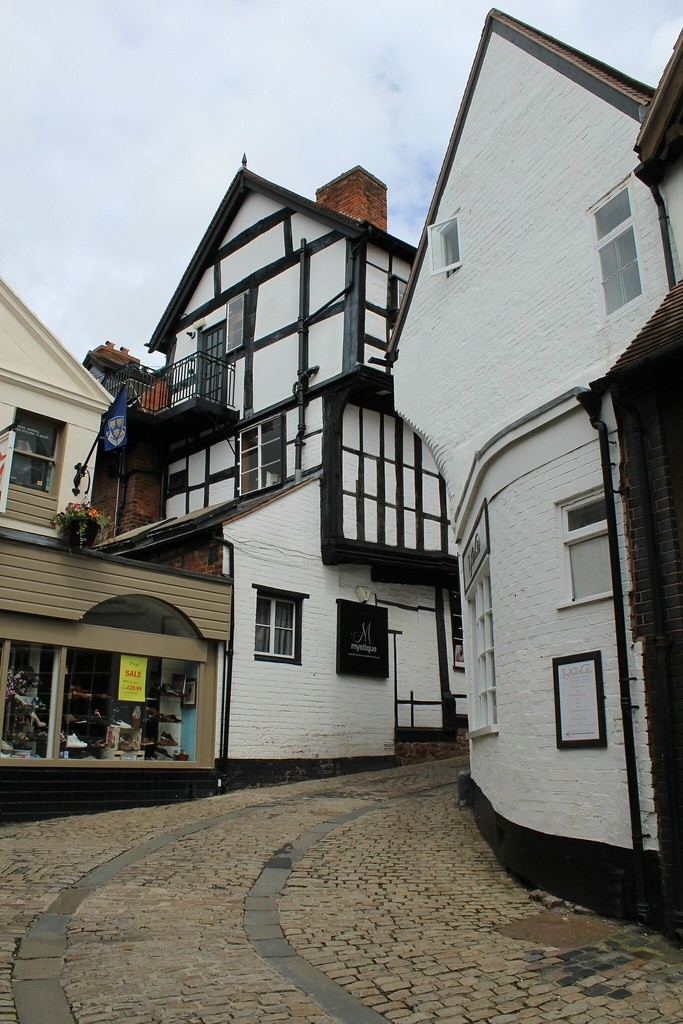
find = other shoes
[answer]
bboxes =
[12,732,30,741]
[63,682,190,761]
[25,754,41,759]
[1,752,11,758]
[0,739,14,750]
[37,729,47,736]
[32,696,46,710]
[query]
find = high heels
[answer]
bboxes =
[13,693,27,706]
[30,711,46,727]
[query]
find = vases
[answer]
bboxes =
[62,517,99,548]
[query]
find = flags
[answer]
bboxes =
[103,388,127,451]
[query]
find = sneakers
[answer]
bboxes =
[65,732,87,748]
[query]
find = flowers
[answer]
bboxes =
[6,665,26,701]
[50,502,118,551]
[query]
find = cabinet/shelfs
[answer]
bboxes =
[9,694,185,752]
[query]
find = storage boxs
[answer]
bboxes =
[106,726,142,750]
[100,749,145,760]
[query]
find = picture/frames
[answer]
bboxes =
[180,678,197,708]
